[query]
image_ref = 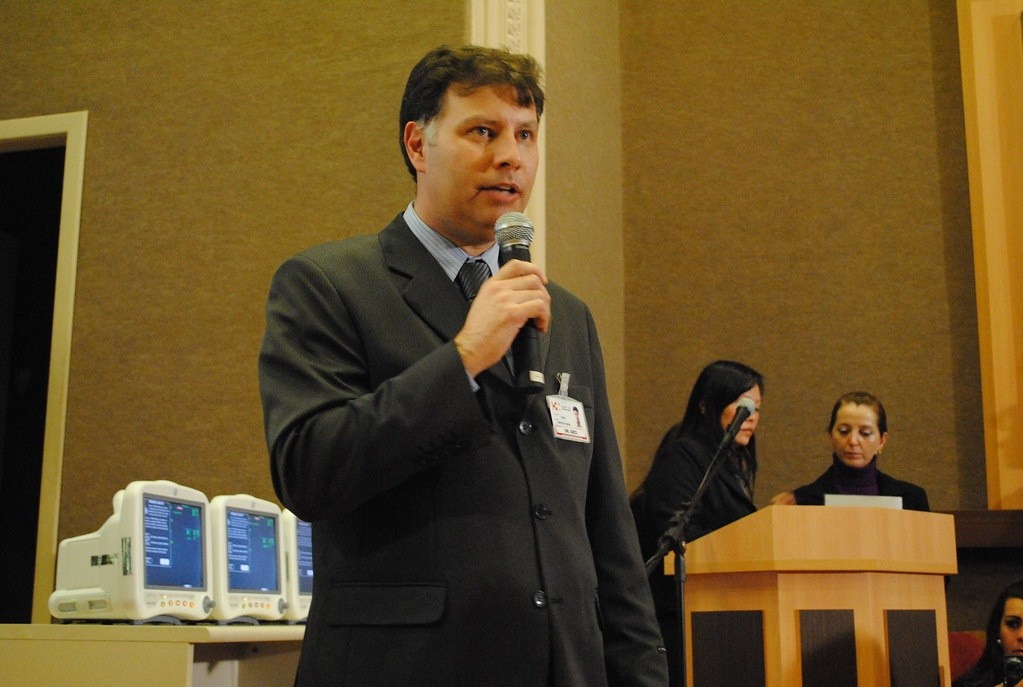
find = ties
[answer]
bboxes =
[457,260,518,382]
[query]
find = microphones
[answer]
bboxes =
[717,397,755,451]
[494,212,546,394]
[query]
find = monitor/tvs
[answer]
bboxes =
[49,479,311,626]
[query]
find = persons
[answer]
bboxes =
[256,45,670,687]
[771,391,931,513]
[951,578,1022,687]
[629,360,765,685]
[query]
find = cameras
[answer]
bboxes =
[1001,654,1023,687]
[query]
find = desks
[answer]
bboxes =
[1,622,309,687]
